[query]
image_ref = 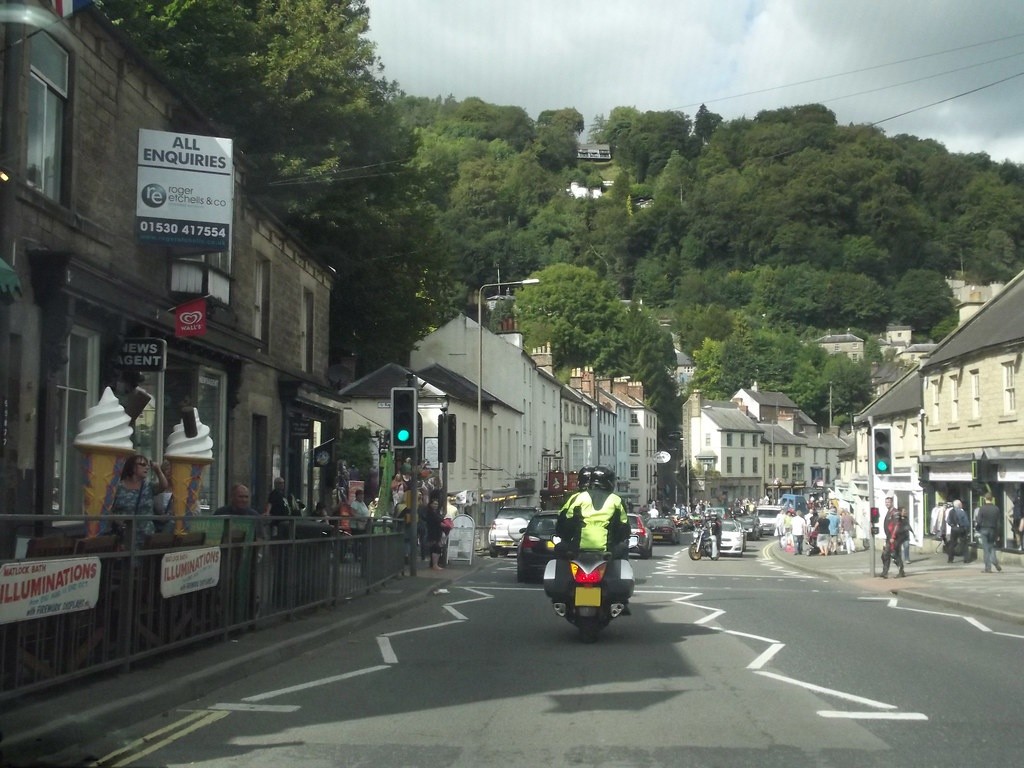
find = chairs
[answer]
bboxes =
[542,519,554,529]
[18,529,248,689]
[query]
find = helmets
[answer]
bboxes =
[579,467,593,491]
[590,464,616,492]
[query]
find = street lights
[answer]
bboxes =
[770,417,793,507]
[686,405,714,514]
[595,375,632,467]
[476,278,540,551]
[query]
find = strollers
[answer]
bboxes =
[804,523,820,556]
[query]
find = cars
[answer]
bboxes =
[641,492,809,561]
[515,508,560,585]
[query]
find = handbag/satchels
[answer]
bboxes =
[112,522,130,562]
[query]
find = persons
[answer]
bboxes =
[777,496,863,556]
[1012,489,1024,550]
[214,477,370,588]
[648,500,722,560]
[556,465,632,616]
[113,454,174,568]
[418,494,446,570]
[947,500,971,563]
[878,497,918,579]
[976,493,1001,573]
[734,497,775,516]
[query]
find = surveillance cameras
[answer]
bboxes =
[920,409,925,416]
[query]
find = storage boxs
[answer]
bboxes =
[543,558,568,602]
[607,558,635,603]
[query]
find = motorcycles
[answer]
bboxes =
[688,517,721,560]
[542,533,640,644]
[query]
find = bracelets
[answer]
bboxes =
[258,553,262,557]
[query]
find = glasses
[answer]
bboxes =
[136,462,148,467]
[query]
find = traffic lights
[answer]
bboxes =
[390,386,417,449]
[870,426,894,476]
[870,507,879,524]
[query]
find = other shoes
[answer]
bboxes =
[981,569,993,573]
[895,572,905,578]
[993,562,1002,571]
[875,572,887,578]
[904,559,911,564]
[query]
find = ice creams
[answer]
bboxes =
[73,386,153,538]
[164,405,215,535]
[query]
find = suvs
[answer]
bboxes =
[487,504,543,558]
[627,512,656,559]
